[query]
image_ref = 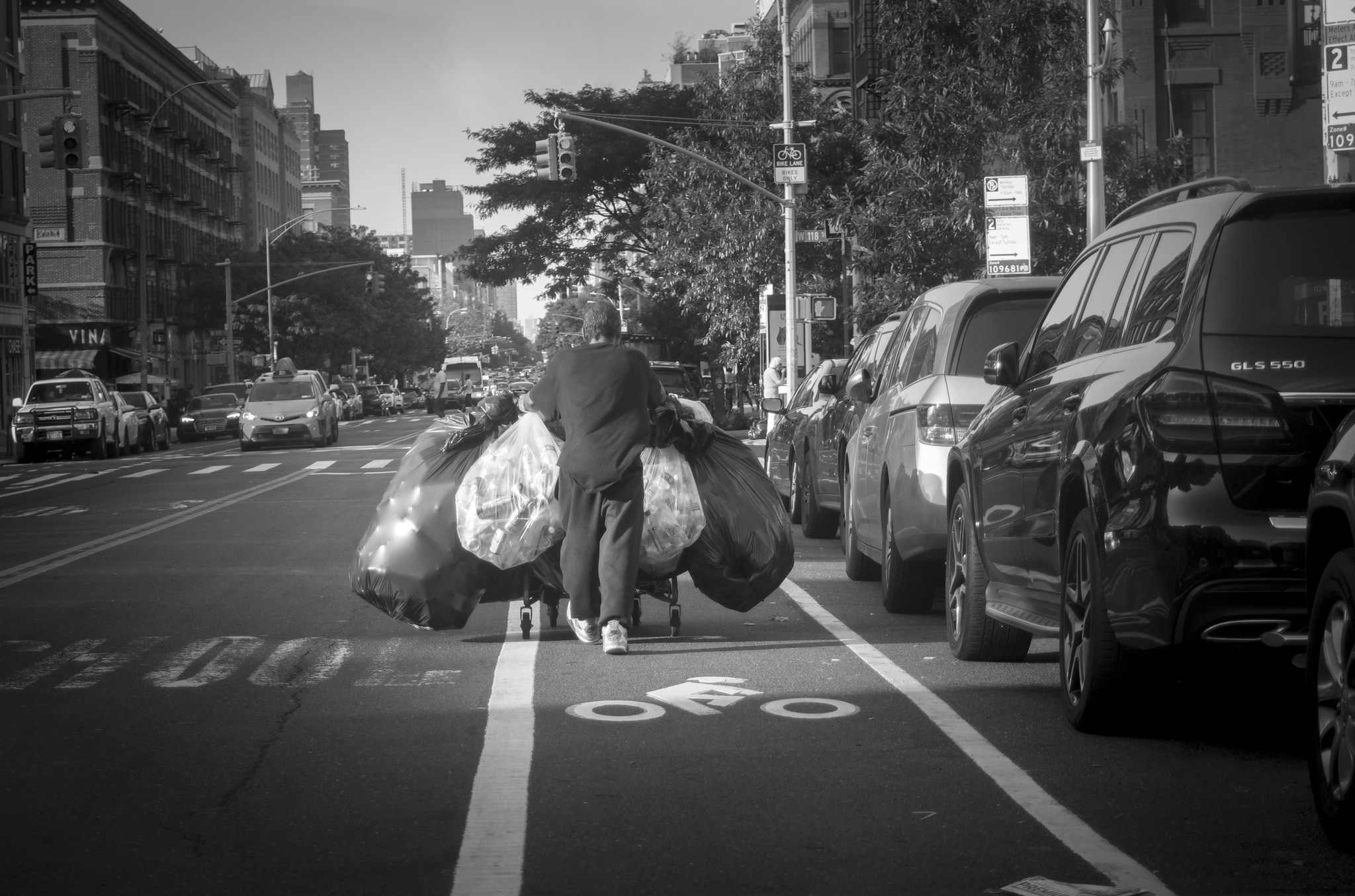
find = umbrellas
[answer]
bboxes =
[114,373,176,385]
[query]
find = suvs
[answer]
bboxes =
[647,311,908,539]
[840,276,1075,613]
[945,175,1353,733]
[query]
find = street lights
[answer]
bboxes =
[137,78,229,398]
[265,206,368,371]
[444,307,468,358]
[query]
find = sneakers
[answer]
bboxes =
[566,600,600,643]
[601,620,629,654]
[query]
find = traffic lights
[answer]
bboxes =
[535,136,555,182]
[811,297,837,321]
[560,338,563,348]
[554,325,559,334]
[54,116,84,171]
[555,337,560,347]
[546,321,552,334]
[557,135,576,181]
[559,287,567,299]
[568,286,578,299]
[495,346,499,354]
[364,273,374,295]
[475,339,484,349]
[491,346,495,355]
[37,121,61,170]
[373,271,385,296]
[425,318,432,332]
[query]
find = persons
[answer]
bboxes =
[457,374,476,413]
[432,361,447,417]
[726,362,760,412]
[763,357,785,437]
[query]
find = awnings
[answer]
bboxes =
[110,347,169,363]
[34,349,101,369]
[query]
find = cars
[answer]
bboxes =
[1299,409,1354,845]
[5,355,550,462]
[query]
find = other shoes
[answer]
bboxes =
[456,410,463,413]
[752,408,755,412]
[470,411,477,414]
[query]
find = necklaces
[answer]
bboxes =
[523,301,671,655]
